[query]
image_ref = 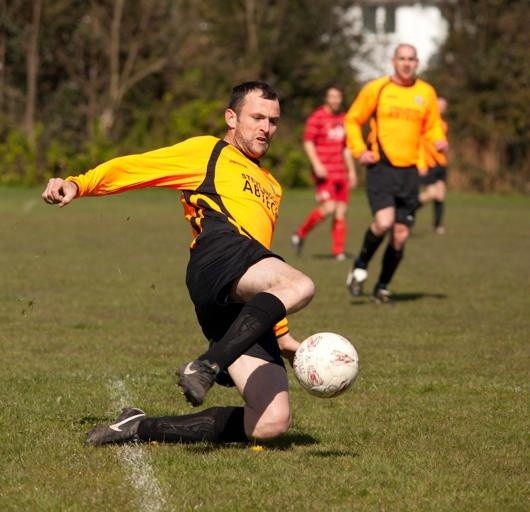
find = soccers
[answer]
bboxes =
[293,332,359,398]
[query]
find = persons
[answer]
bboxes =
[41,81,316,449]
[345,44,449,305]
[292,82,356,263]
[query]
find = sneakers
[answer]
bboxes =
[430,226,446,234]
[292,233,304,257]
[85,408,147,447]
[373,288,395,303]
[337,253,345,261]
[346,268,368,295]
[175,358,216,407]
